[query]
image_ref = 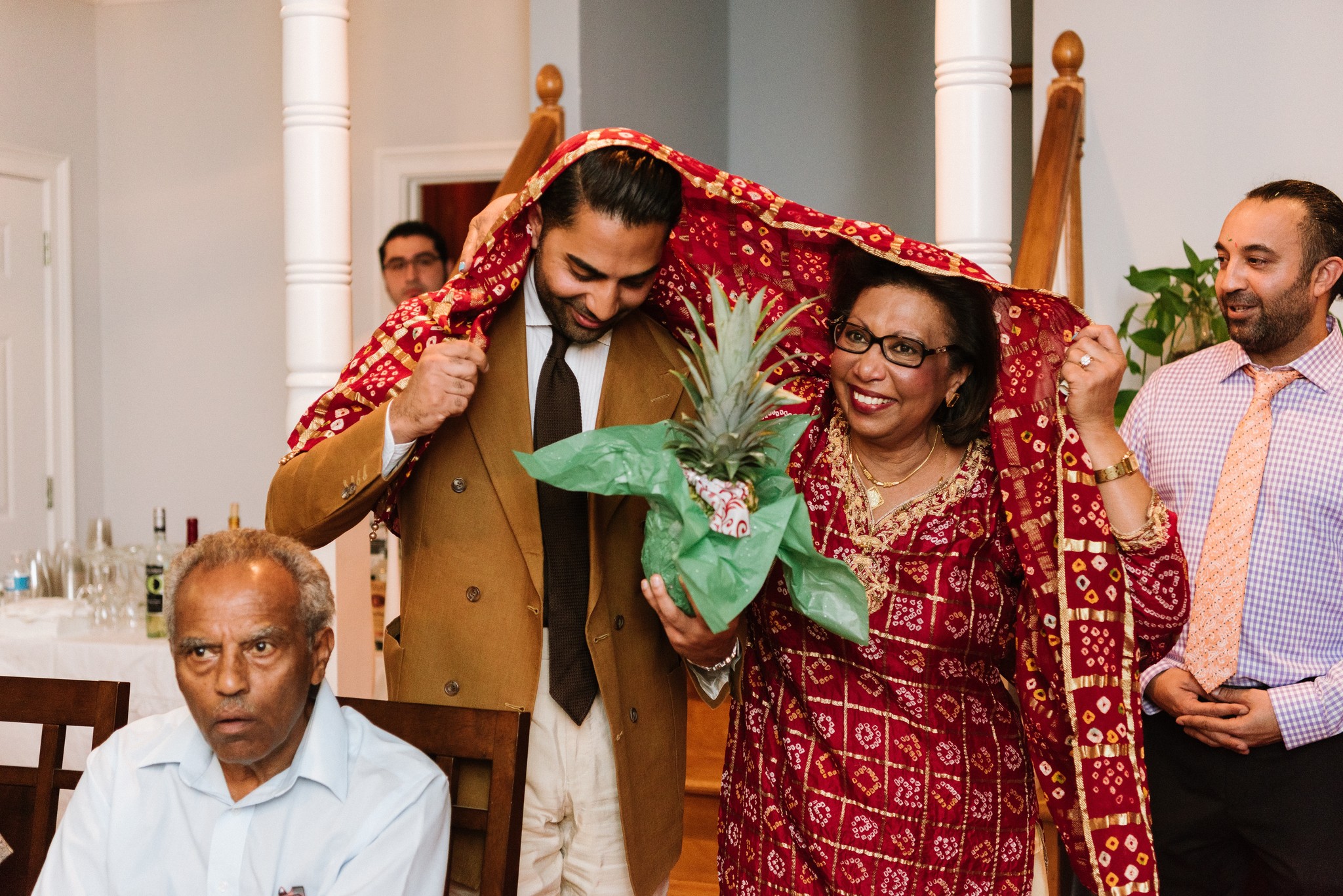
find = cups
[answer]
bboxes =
[5,529,146,635]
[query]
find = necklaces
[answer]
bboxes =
[848,425,946,547]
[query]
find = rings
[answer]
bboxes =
[1079,354,1095,368]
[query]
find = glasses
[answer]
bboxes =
[382,253,444,273]
[829,313,961,369]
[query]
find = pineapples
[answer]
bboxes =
[662,273,827,518]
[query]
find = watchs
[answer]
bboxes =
[1094,450,1139,484]
[702,640,738,672]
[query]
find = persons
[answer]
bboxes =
[379,220,455,306]
[462,193,1192,896]
[33,528,452,896]
[265,146,744,896]
[1118,179,1343,896]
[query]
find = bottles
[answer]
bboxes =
[146,508,176,639]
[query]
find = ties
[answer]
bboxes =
[1185,362,1304,693]
[534,324,600,727]
